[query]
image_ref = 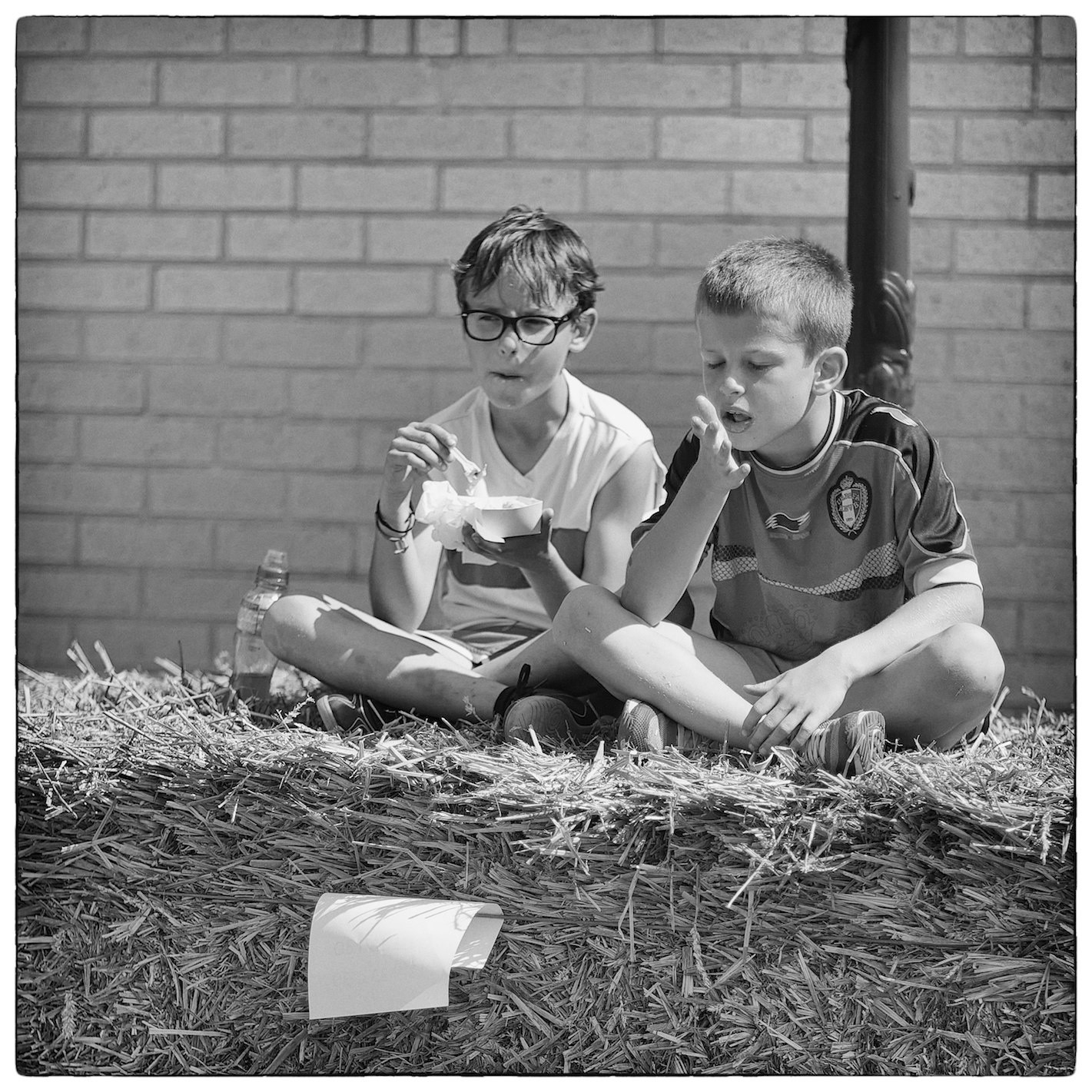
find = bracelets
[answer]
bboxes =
[375,498,416,533]
[375,509,413,555]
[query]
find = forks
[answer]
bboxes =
[449,446,480,479]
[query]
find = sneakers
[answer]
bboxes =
[618,697,723,766]
[789,710,886,777]
[316,692,412,736]
[493,663,617,751]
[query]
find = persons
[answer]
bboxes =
[261,208,695,750]
[553,238,1005,776]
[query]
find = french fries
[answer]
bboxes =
[466,463,487,496]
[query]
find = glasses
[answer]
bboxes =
[461,300,579,346]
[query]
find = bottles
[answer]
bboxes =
[231,548,292,689]
